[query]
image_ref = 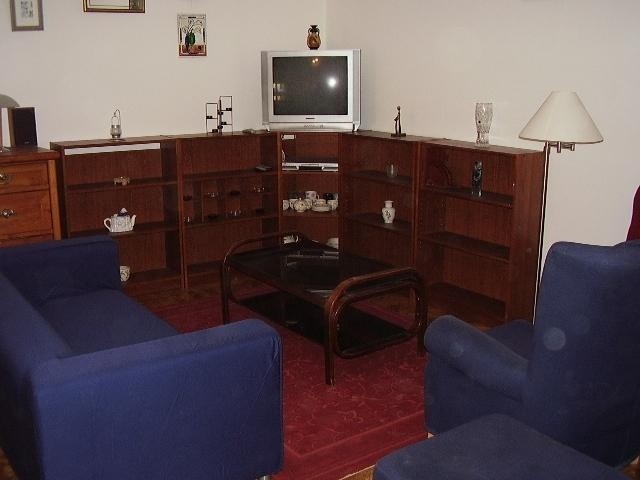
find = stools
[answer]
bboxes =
[373,412,631,480]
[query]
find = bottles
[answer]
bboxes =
[293,197,309,212]
[306,24,321,50]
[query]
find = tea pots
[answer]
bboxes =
[103,207,137,233]
[283,233,299,244]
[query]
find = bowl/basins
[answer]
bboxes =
[311,204,332,212]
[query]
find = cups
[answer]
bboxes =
[289,198,298,210]
[386,161,398,177]
[474,102,493,148]
[120,266,130,282]
[283,199,289,211]
[304,199,312,210]
[315,198,326,205]
[327,200,338,211]
[305,190,319,205]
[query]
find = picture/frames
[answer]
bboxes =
[83,0,144,13]
[10,0,43,30]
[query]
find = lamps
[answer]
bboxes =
[519,88,603,324]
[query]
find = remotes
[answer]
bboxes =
[243,128,256,134]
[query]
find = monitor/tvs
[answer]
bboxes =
[261,49,361,133]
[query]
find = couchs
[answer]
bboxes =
[0,235,284,480]
[424,240,640,471]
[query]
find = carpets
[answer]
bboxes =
[151,285,427,480]
